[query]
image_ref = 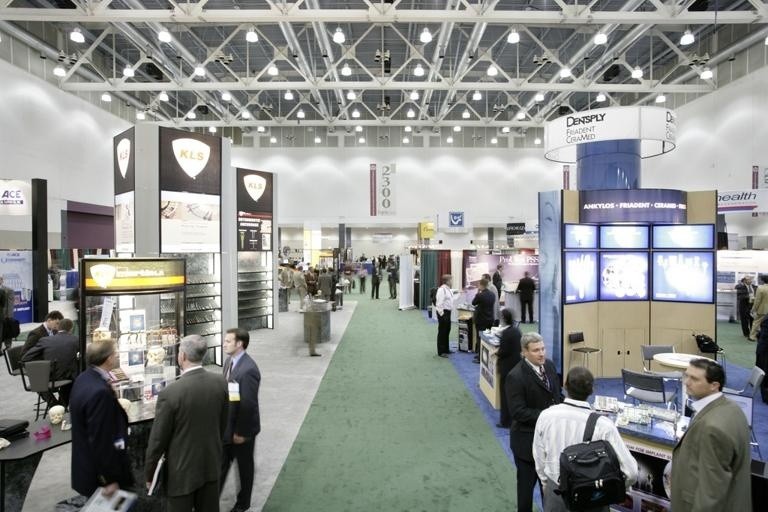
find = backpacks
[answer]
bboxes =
[430,287,446,306]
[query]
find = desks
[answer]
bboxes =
[0,399,156,511]
[653,353,720,415]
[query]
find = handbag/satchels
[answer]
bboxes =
[558,439,628,512]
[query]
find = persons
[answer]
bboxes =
[735,274,768,341]
[70,339,136,498]
[143,334,230,512]
[435,264,536,428]
[504,331,565,512]
[20,319,79,412]
[278,253,400,357]
[18,311,64,360]
[219,328,261,511]
[532,367,639,511]
[668,359,754,512]
[0,278,15,356]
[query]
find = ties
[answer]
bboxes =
[540,366,550,390]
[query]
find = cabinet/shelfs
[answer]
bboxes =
[480,332,504,411]
[237,250,274,330]
[79,258,186,399]
[649,301,717,378]
[161,251,220,366]
[598,300,651,379]
[563,300,600,388]
[457,309,478,352]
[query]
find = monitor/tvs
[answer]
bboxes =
[599,251,649,301]
[563,252,597,304]
[652,251,714,304]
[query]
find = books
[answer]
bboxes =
[147,453,166,496]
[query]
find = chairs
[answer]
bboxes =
[18,360,73,422]
[568,332,603,378]
[3,347,30,376]
[640,344,676,377]
[692,334,726,368]
[621,369,679,411]
[686,366,765,459]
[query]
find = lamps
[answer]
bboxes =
[54,26,717,119]
